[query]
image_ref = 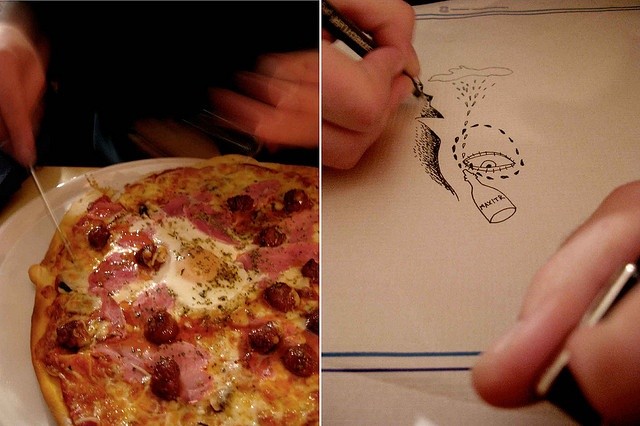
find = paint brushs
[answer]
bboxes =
[322,0,422,97]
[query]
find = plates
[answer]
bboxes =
[0,156,206,425]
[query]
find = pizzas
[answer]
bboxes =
[0,154,319,426]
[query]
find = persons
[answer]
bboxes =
[322,0,419,170]
[0,1,319,165]
[471,178,640,426]
